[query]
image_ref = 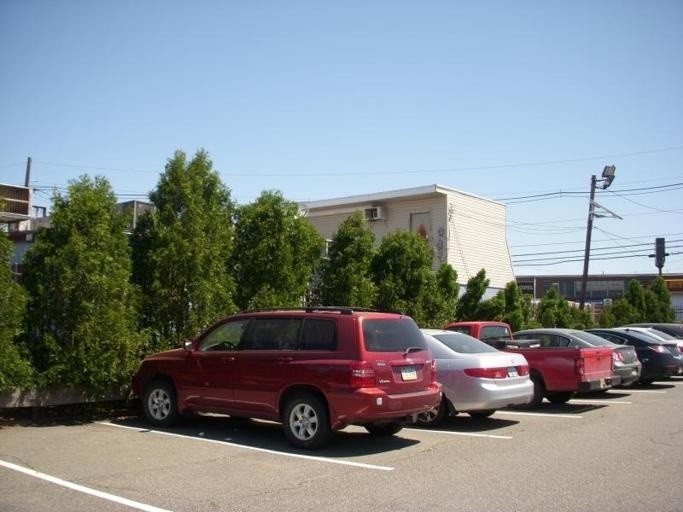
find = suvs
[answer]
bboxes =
[134,307,443,450]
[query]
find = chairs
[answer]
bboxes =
[252,329,278,349]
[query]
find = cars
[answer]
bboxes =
[511,329,643,388]
[610,326,681,356]
[418,329,535,428]
[582,328,681,388]
[619,323,681,340]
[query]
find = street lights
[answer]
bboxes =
[578,165,616,309]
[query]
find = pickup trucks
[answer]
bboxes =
[442,322,614,410]
